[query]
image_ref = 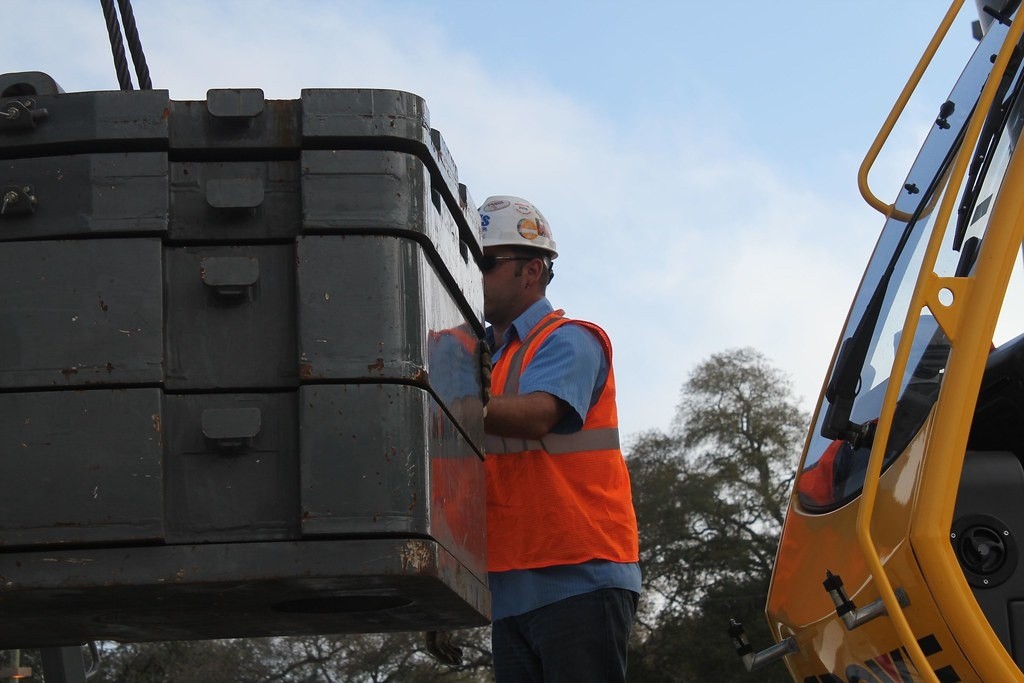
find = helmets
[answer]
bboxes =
[477,196,558,260]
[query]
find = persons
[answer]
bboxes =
[426,323,486,575]
[477,196,642,683]
[799,417,878,506]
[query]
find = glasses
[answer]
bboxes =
[484,254,534,271]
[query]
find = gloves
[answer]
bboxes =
[481,340,491,418]
